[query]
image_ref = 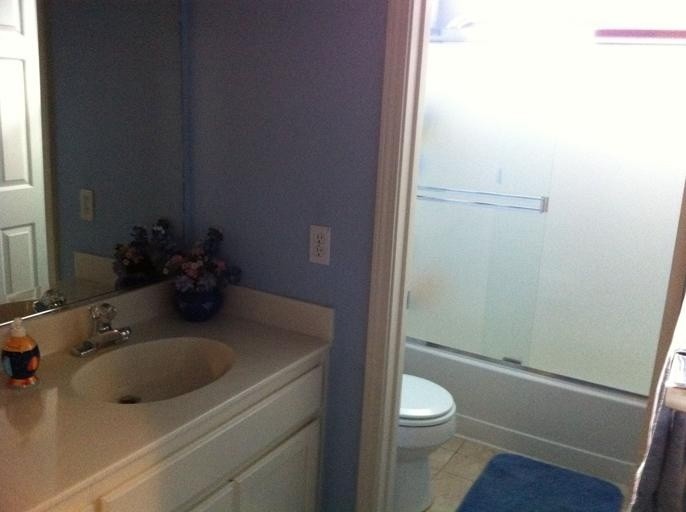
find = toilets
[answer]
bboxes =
[398,373,457,511]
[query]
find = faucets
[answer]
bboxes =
[71,303,131,355]
[33,289,65,311]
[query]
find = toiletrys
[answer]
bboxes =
[1,319,42,387]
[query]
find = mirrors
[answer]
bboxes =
[0,0,186,327]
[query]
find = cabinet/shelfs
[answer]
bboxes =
[92,364,326,512]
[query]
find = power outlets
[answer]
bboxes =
[308,225,332,266]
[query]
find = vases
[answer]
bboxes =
[175,290,224,323]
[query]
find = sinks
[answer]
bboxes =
[1,300,35,322]
[72,337,234,405]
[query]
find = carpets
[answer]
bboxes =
[452,451,625,511]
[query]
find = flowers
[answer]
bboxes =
[161,227,243,296]
[113,218,170,287]
